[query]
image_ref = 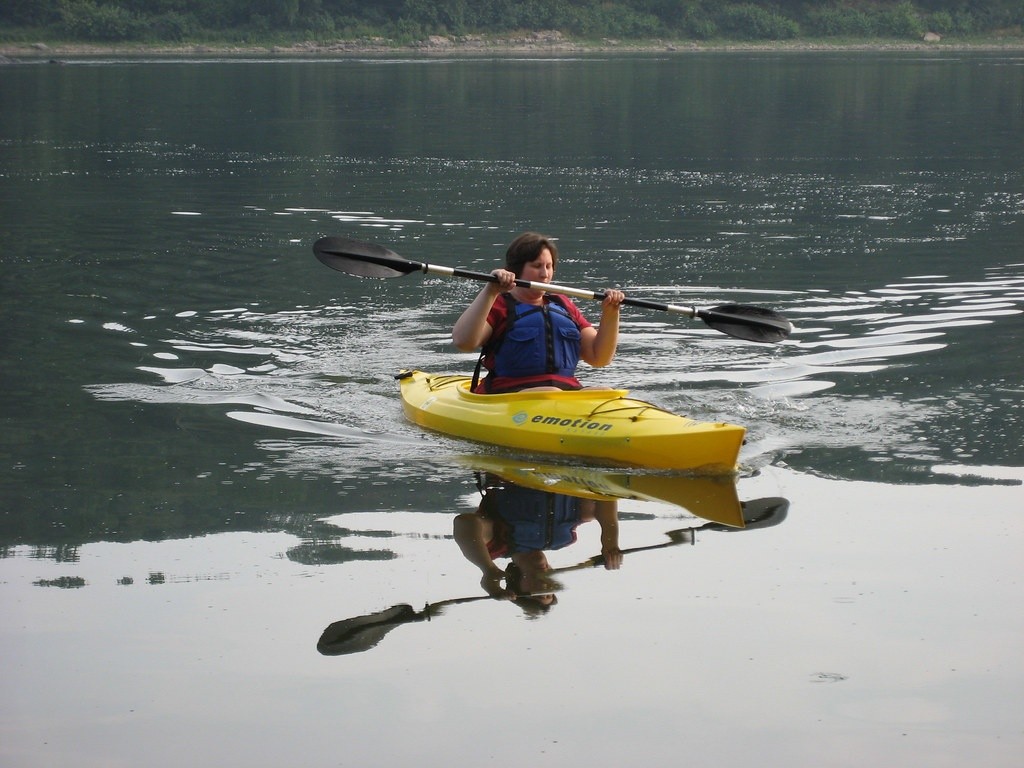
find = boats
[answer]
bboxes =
[393,364,751,476]
[453,454,749,533]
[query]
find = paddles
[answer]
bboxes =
[314,495,790,658]
[309,233,794,345]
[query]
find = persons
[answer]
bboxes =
[453,473,624,614]
[451,232,625,391]
